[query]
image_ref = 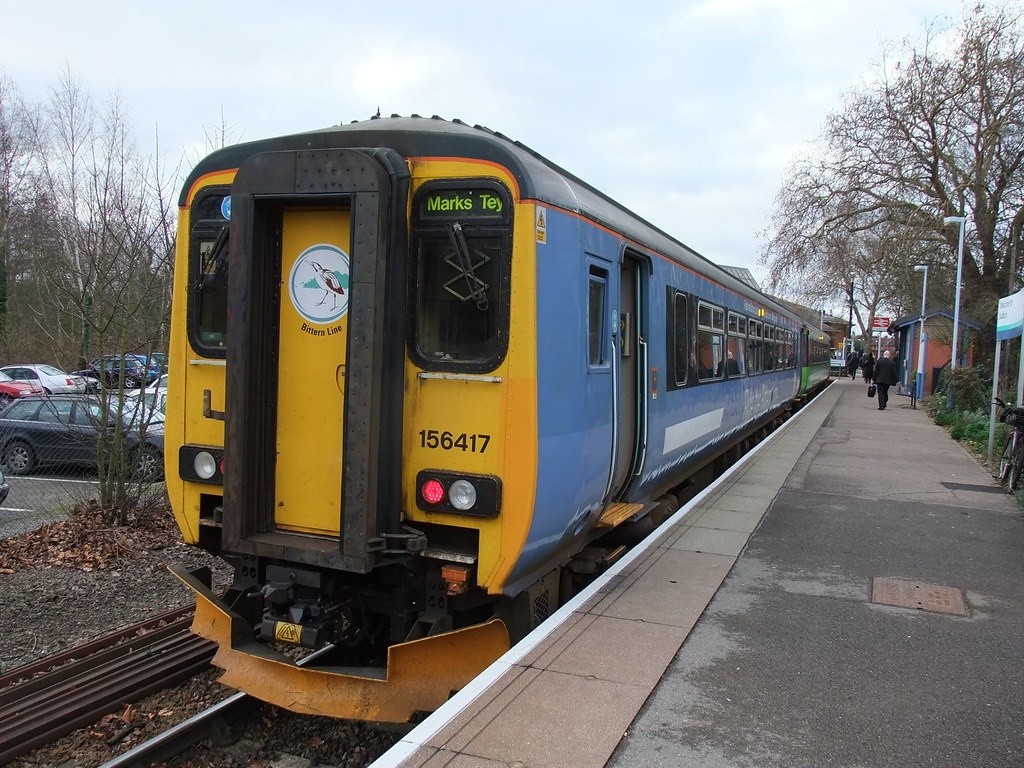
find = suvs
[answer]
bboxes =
[0,388,165,483]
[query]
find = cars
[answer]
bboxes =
[0,363,104,395]
[71,351,168,417]
[0,370,46,413]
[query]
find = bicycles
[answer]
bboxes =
[993,396,1024,495]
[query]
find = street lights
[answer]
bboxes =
[913,265,929,399]
[847,271,856,355]
[943,216,966,410]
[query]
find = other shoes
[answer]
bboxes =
[878,407,886,410]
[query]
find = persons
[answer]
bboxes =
[872,350,900,410]
[892,351,899,382]
[846,350,875,383]
[717,352,739,377]
[787,354,792,366]
[831,354,836,359]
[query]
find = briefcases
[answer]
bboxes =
[867,383,876,397]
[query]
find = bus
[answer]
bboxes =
[164,105,836,724]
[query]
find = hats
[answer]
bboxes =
[883,350,890,356]
